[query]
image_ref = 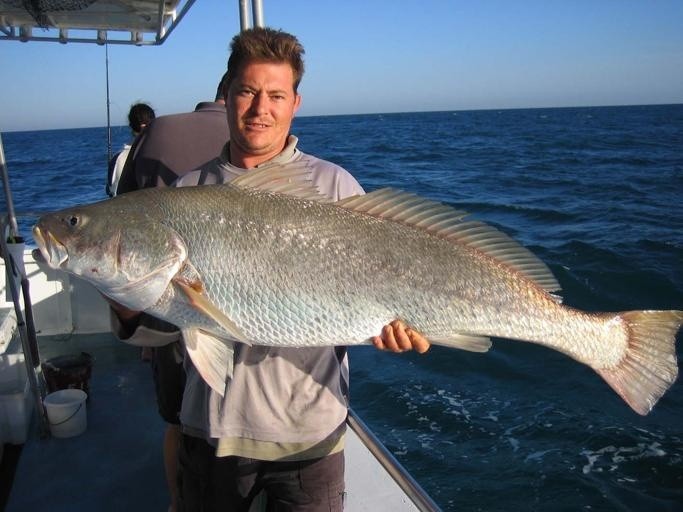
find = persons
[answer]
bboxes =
[104,102,163,364]
[96,24,429,512]
[115,69,232,512]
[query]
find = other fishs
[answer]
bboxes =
[30,159,683,417]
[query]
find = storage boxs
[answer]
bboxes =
[0,352,40,445]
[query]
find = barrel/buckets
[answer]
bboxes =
[41,351,97,406]
[42,387,89,440]
